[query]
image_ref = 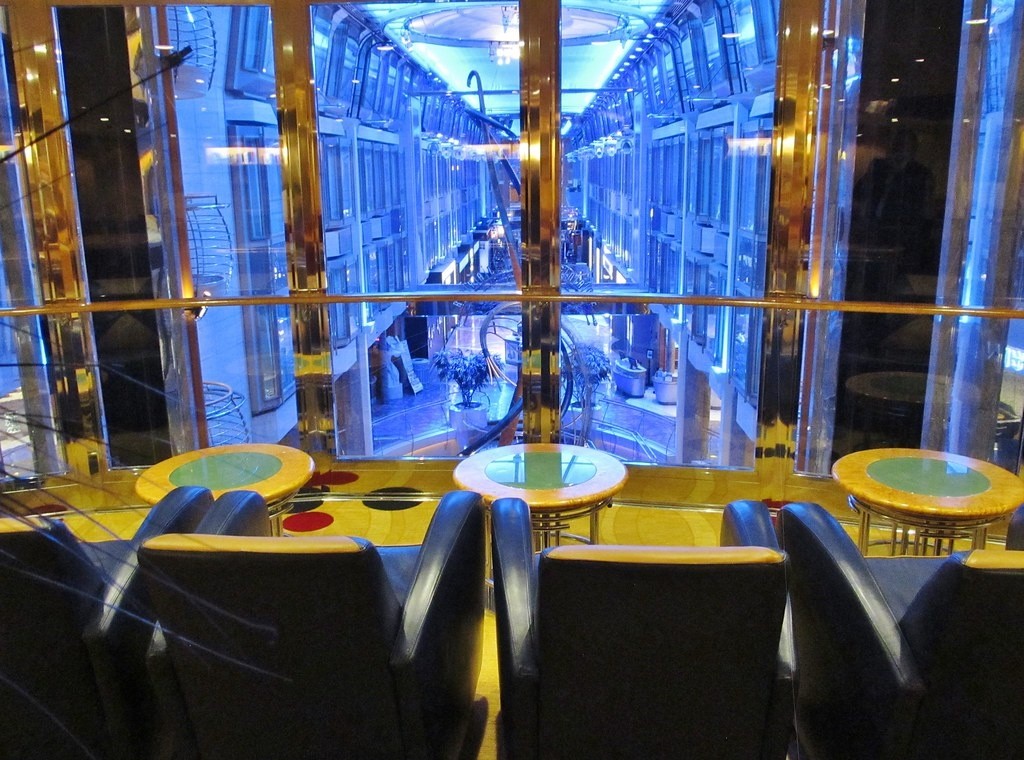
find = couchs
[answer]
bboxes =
[0,485,213,760]
[775,502,1024,760]
[137,490,488,760]
[491,499,790,760]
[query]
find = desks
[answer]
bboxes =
[133,443,316,537]
[452,444,629,612]
[831,448,1024,556]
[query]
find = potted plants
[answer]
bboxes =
[560,342,612,430]
[433,348,506,447]
